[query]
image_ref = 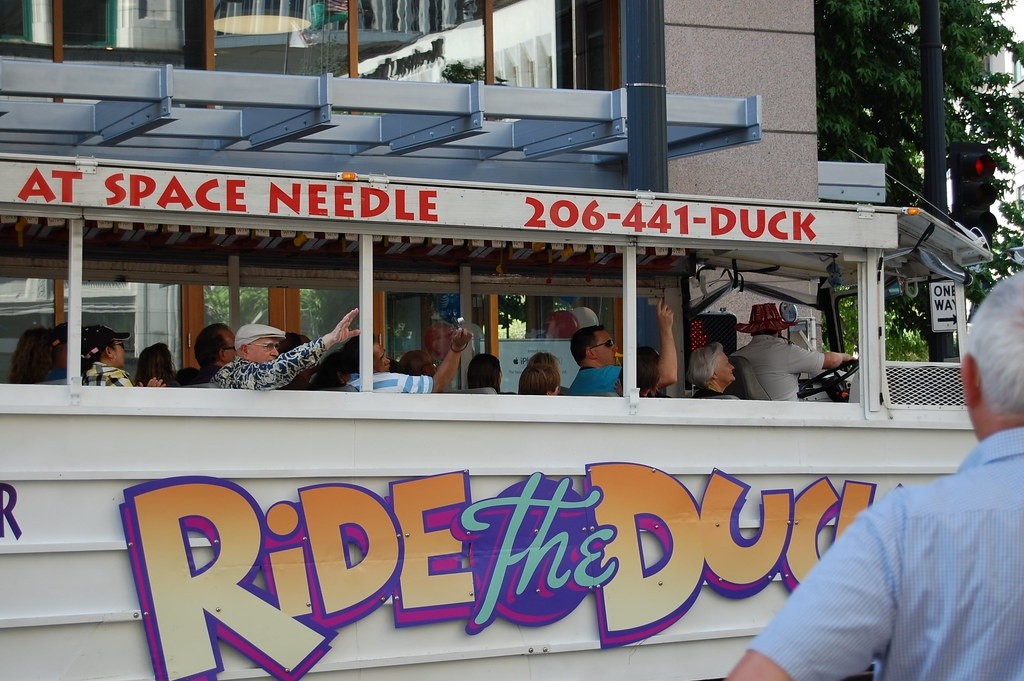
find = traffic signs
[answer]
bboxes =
[928,280,957,332]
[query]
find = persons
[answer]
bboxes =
[467,353,517,394]
[686,342,744,400]
[730,303,856,401]
[725,268,1024,681]
[519,352,561,395]
[566,324,622,395]
[9,307,472,393]
[619,298,677,398]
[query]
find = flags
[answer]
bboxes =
[328,0,349,11]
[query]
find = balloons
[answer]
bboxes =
[573,307,599,329]
[544,310,578,338]
[423,293,460,361]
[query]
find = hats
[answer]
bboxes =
[49,322,67,347]
[235,324,287,349]
[735,303,798,331]
[81,325,131,358]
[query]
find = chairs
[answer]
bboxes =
[728,354,771,401]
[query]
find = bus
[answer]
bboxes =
[0,151,994,681]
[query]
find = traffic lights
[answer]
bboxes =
[942,140,1000,249]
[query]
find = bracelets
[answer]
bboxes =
[451,343,468,352]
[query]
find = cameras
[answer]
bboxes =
[451,316,465,330]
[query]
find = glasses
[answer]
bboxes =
[250,341,279,351]
[421,362,437,370]
[108,341,125,349]
[589,339,615,349]
